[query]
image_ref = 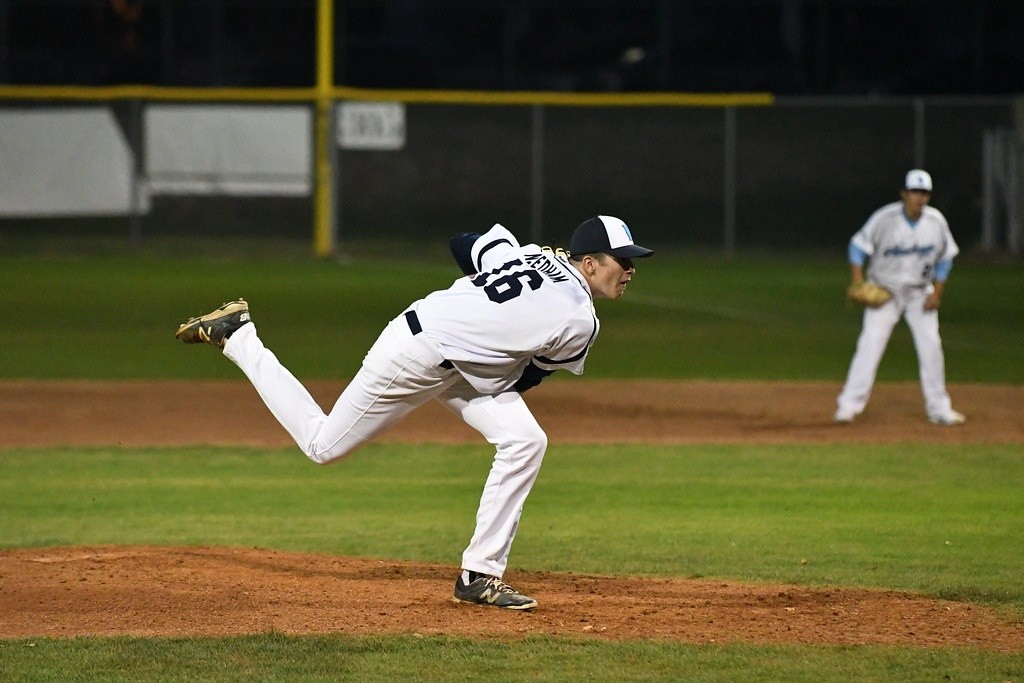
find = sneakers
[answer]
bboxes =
[831,405,855,423]
[454,573,538,612]
[172,297,253,344]
[930,407,967,425]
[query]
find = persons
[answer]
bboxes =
[825,166,971,426]
[170,212,655,612]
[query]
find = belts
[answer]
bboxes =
[404,296,456,370]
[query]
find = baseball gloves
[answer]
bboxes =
[845,276,892,309]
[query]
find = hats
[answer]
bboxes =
[570,215,654,262]
[905,167,934,197]
